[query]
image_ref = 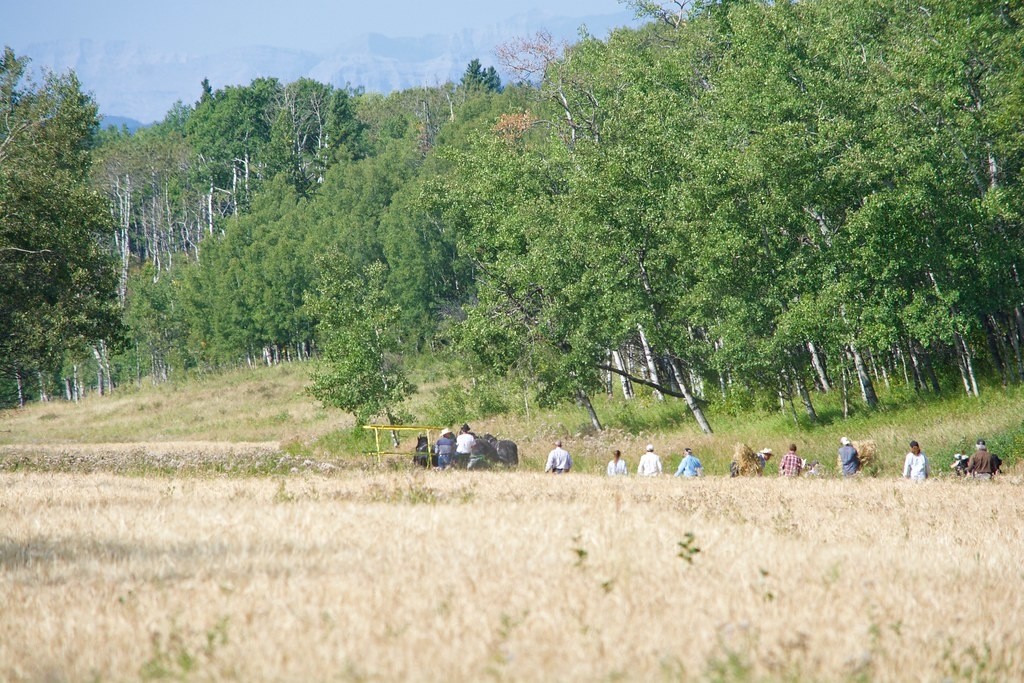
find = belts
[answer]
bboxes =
[977,472,990,474]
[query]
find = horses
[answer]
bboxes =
[413,432,518,471]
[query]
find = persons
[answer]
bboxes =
[780,443,802,477]
[730,448,775,477]
[956,439,1002,480]
[903,441,930,480]
[435,423,476,470]
[608,450,627,476]
[638,445,663,477]
[839,438,860,477]
[545,440,573,473]
[674,448,702,477]
[805,462,819,477]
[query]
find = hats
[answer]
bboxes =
[555,440,562,445]
[910,441,918,448]
[760,448,774,456]
[463,424,470,432]
[977,439,985,445]
[440,428,452,436]
[840,437,850,444]
[685,448,692,453]
[646,444,654,451]
